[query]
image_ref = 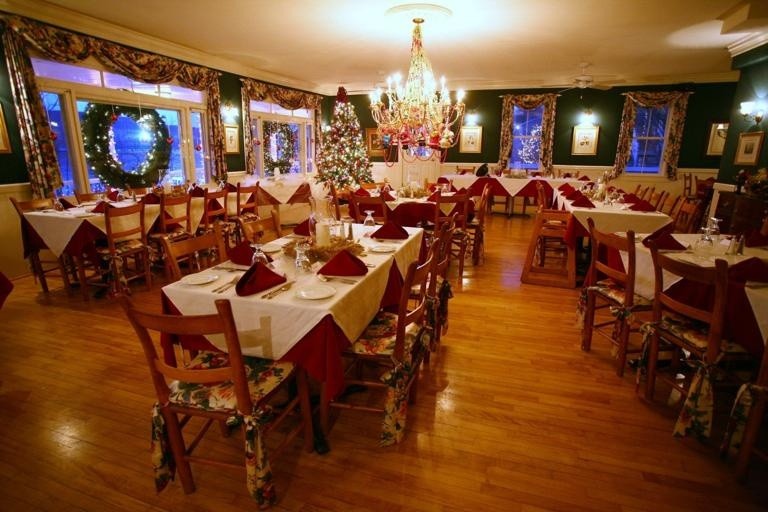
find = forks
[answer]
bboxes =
[316,273,355,285]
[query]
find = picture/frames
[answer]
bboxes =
[733,132,765,167]
[224,124,240,155]
[706,122,731,157]
[572,126,599,155]
[460,126,482,154]
[365,127,388,157]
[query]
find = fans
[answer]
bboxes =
[539,61,627,94]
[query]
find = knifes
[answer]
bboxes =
[262,281,295,299]
[214,266,248,272]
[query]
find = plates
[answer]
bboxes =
[298,284,336,299]
[368,245,395,252]
[615,231,639,240]
[182,272,219,285]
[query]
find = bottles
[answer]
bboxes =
[308,195,339,245]
[725,236,736,256]
[157,167,174,195]
[549,172,555,179]
[736,235,745,255]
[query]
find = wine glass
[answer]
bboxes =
[115,188,125,204]
[691,216,724,259]
[184,180,202,193]
[219,180,224,190]
[249,243,268,267]
[373,182,449,198]
[578,178,624,208]
[52,195,64,212]
[260,210,375,273]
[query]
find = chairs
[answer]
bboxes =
[436,163,594,219]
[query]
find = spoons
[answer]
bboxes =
[268,284,292,300]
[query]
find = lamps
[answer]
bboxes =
[369,3,467,167]
[739,99,767,125]
